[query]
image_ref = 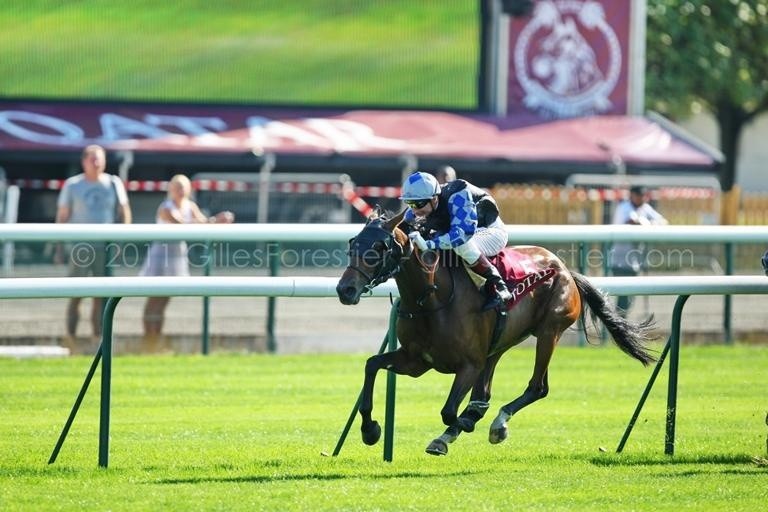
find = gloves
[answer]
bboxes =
[408,231,428,252]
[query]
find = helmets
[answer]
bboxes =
[398,172,442,200]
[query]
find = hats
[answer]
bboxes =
[630,184,647,196]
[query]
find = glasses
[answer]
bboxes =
[404,199,431,210]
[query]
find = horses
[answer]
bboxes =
[335,203,667,456]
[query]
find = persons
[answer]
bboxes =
[51,143,134,338]
[393,171,512,312]
[435,165,459,185]
[137,171,232,336]
[604,181,672,320]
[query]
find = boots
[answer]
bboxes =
[469,254,513,311]
